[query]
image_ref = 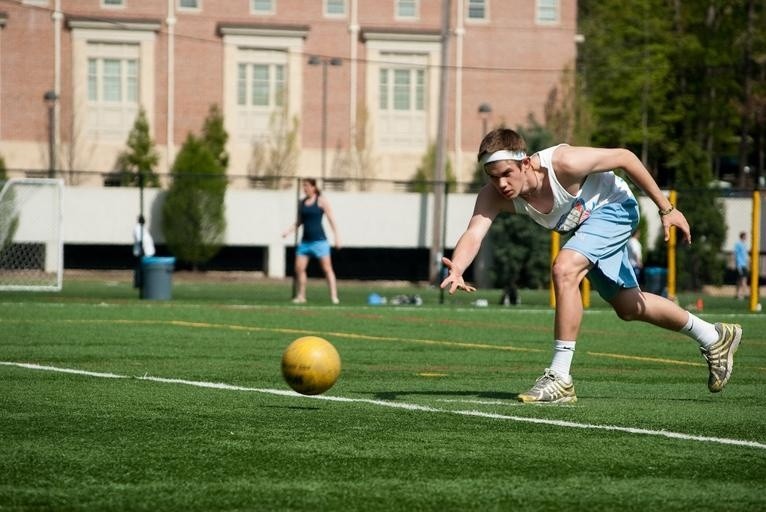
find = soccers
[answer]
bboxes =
[281,335,341,396]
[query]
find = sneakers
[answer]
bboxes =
[517,367,577,404]
[700,322,743,393]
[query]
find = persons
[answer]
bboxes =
[132,213,159,290]
[628,227,644,277]
[439,128,745,405]
[282,176,341,304]
[732,230,750,300]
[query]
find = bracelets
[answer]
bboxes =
[659,202,675,216]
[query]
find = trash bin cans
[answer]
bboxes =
[141,257,175,299]
[644,267,667,296]
